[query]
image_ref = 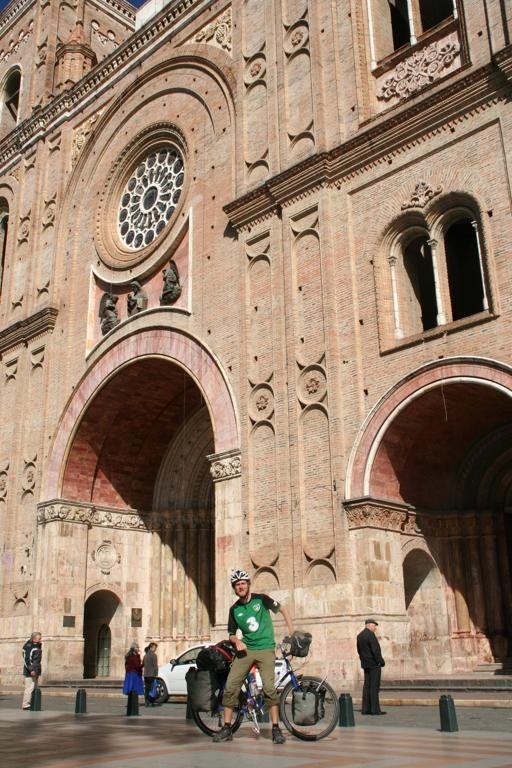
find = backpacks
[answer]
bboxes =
[185,640,238,712]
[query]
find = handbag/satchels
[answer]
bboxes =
[148,680,159,699]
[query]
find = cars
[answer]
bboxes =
[143,642,291,706]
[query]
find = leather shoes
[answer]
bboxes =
[23,707,30,710]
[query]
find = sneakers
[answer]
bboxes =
[272,727,286,744]
[213,725,232,742]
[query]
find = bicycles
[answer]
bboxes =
[188,636,340,741]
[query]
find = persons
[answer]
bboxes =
[213,570,294,743]
[127,281,148,317]
[123,642,144,695]
[143,643,158,707]
[22,632,42,710]
[162,259,180,291]
[99,293,119,324]
[356,619,387,715]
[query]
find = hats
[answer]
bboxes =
[365,618,378,625]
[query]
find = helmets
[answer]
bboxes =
[230,568,250,583]
